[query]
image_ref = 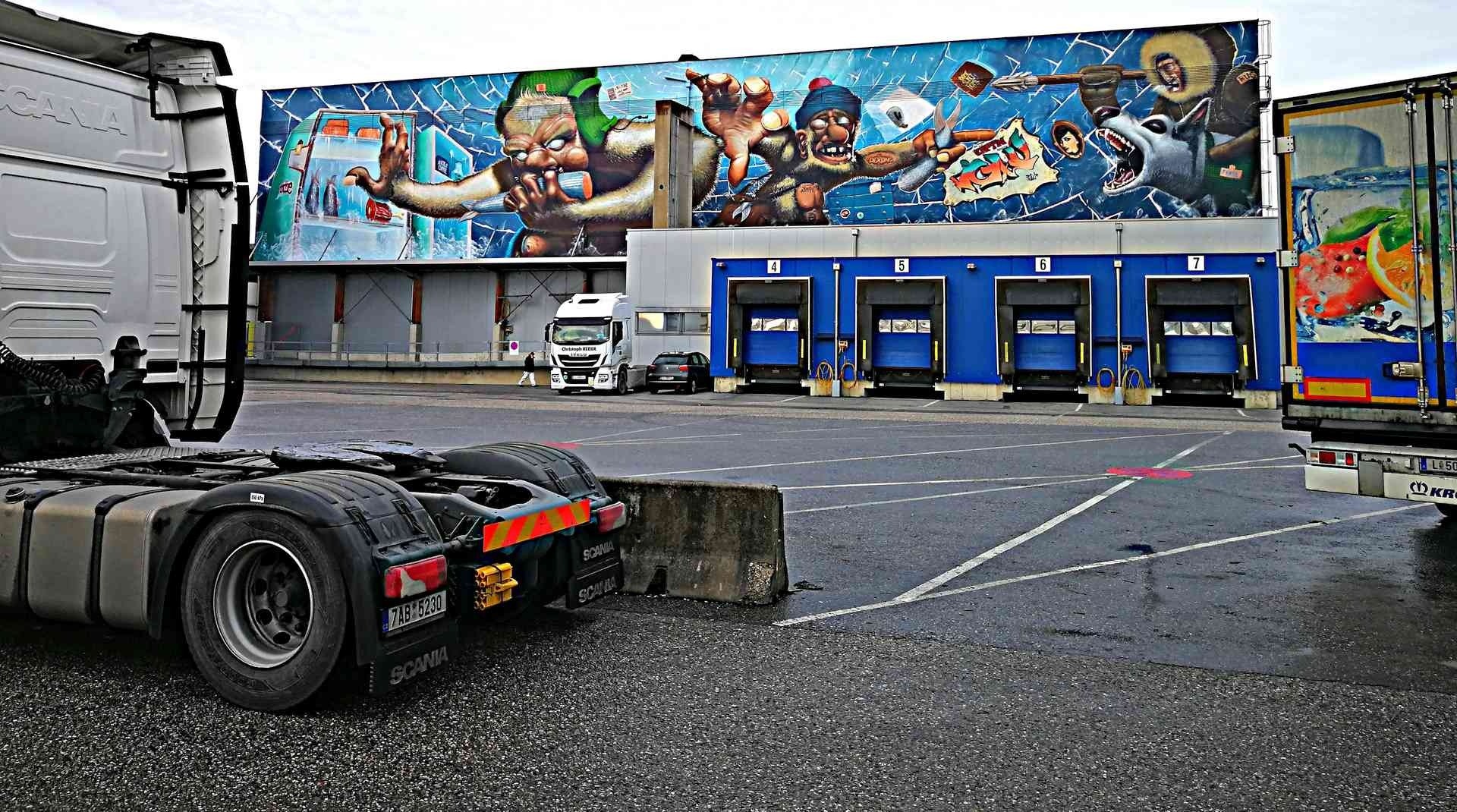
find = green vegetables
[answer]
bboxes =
[1316,187,1457,266]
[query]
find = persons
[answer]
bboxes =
[519,352,540,387]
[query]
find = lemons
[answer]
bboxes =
[1365,225,1456,312]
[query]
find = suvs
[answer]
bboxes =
[646,351,710,395]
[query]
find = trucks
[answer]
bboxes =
[1273,74,1457,515]
[544,293,645,396]
[0,0,629,716]
